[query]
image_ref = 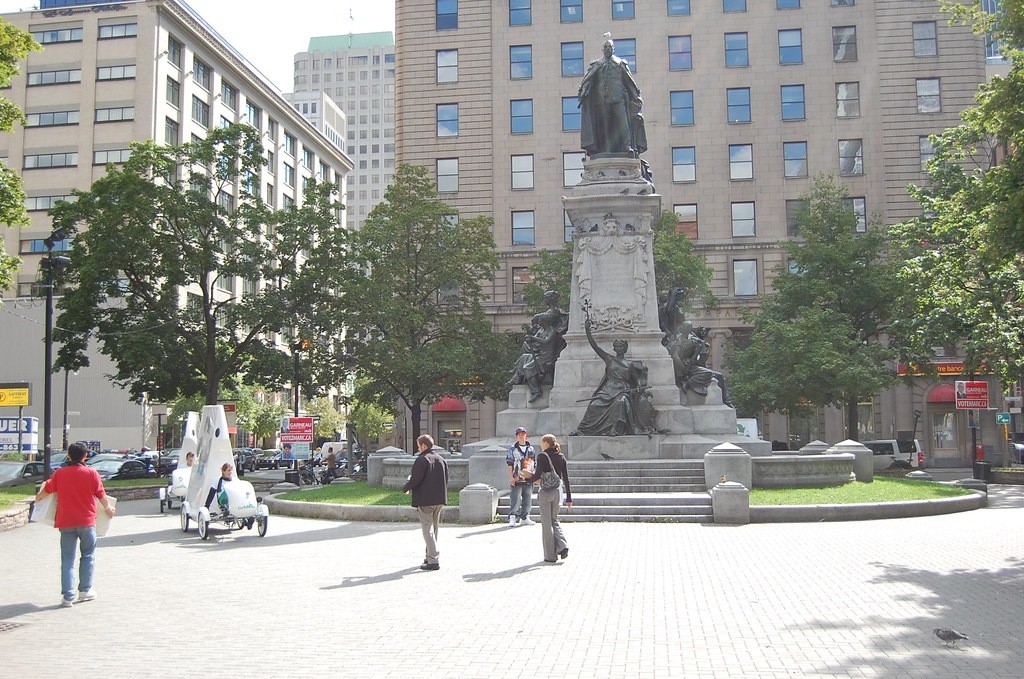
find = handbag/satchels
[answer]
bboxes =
[536,453,560,491]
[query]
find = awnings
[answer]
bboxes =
[926,383,955,402]
[432,395,466,411]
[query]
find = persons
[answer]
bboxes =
[568,320,642,436]
[577,40,643,156]
[321,447,340,485]
[181,451,194,468]
[957,382,966,399]
[283,444,296,459]
[402,435,448,569]
[36,442,115,607]
[281,417,289,433]
[314,447,322,460]
[504,291,568,401]
[217,462,254,530]
[505,427,535,526]
[523,435,573,562]
[666,320,734,408]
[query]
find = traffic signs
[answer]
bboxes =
[996,412,1011,424]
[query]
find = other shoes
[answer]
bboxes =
[423,558,428,564]
[78,592,94,601]
[421,564,440,570]
[247,517,254,530]
[61,598,72,607]
[544,559,556,562]
[561,549,568,559]
[224,510,229,517]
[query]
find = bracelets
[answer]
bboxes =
[104,505,111,510]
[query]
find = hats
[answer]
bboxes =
[515,427,526,434]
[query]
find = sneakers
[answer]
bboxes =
[519,516,536,525]
[509,515,516,526]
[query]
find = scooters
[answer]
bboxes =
[332,455,368,478]
[298,461,319,485]
[317,464,334,485]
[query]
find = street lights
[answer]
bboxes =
[43,227,74,481]
[293,339,311,417]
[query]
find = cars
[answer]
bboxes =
[0,460,56,489]
[85,445,182,478]
[255,448,293,470]
[231,446,263,472]
[50,449,99,470]
[88,457,158,481]
[307,447,322,468]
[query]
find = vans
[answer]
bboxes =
[321,441,362,462]
[860,439,924,472]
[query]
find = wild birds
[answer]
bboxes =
[933,628,968,646]
[720,474,727,482]
[601,453,615,461]
